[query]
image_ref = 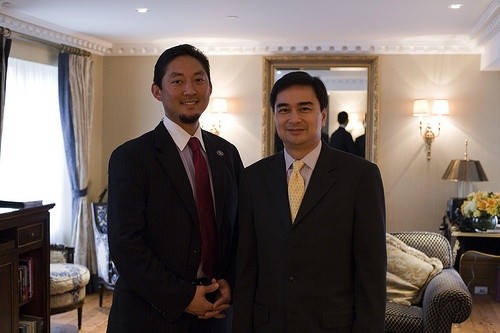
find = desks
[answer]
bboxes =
[444,218,500,266]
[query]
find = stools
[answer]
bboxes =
[49,264,90,330]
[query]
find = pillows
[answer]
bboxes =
[386,232,443,306]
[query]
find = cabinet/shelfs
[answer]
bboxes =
[0,200,56,333]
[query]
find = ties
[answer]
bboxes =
[188,137,219,282]
[288,160,306,224]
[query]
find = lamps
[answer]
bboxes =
[413,99,449,162]
[207,98,228,133]
[439,138,489,196]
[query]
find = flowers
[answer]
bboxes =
[460,191,500,219]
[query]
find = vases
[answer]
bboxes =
[473,214,498,232]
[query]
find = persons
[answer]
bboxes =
[244,71,387,333]
[330,111,366,157]
[107,44,245,333]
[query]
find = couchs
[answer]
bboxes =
[386,231,474,333]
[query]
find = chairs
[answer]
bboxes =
[90,202,118,308]
